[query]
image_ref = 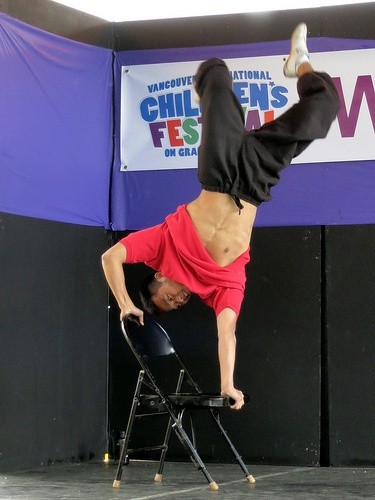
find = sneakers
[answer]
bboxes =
[282,23,311,78]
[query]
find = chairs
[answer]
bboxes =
[111,313,256,491]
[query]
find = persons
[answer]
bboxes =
[100,20,340,409]
